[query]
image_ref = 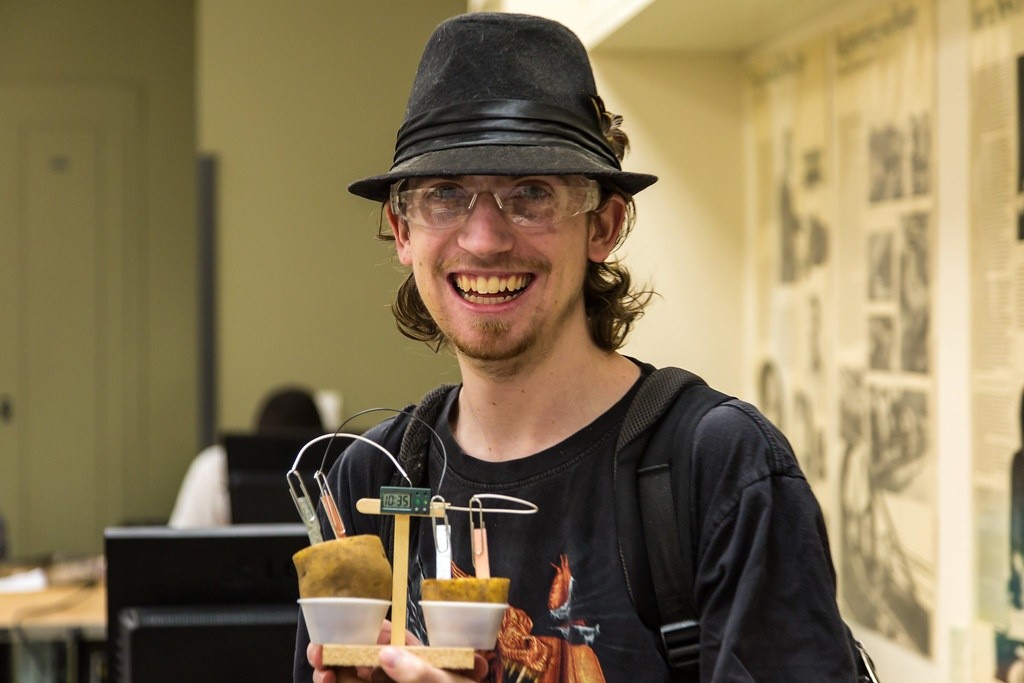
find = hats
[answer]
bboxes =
[347,11,658,205]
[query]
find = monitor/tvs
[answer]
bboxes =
[222,430,364,526]
[101,523,321,683]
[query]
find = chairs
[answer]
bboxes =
[220,429,363,523]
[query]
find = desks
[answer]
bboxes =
[0,577,109,683]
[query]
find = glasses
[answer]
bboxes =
[389,175,601,227]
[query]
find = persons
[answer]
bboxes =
[167,385,324,530]
[292,12,858,683]
